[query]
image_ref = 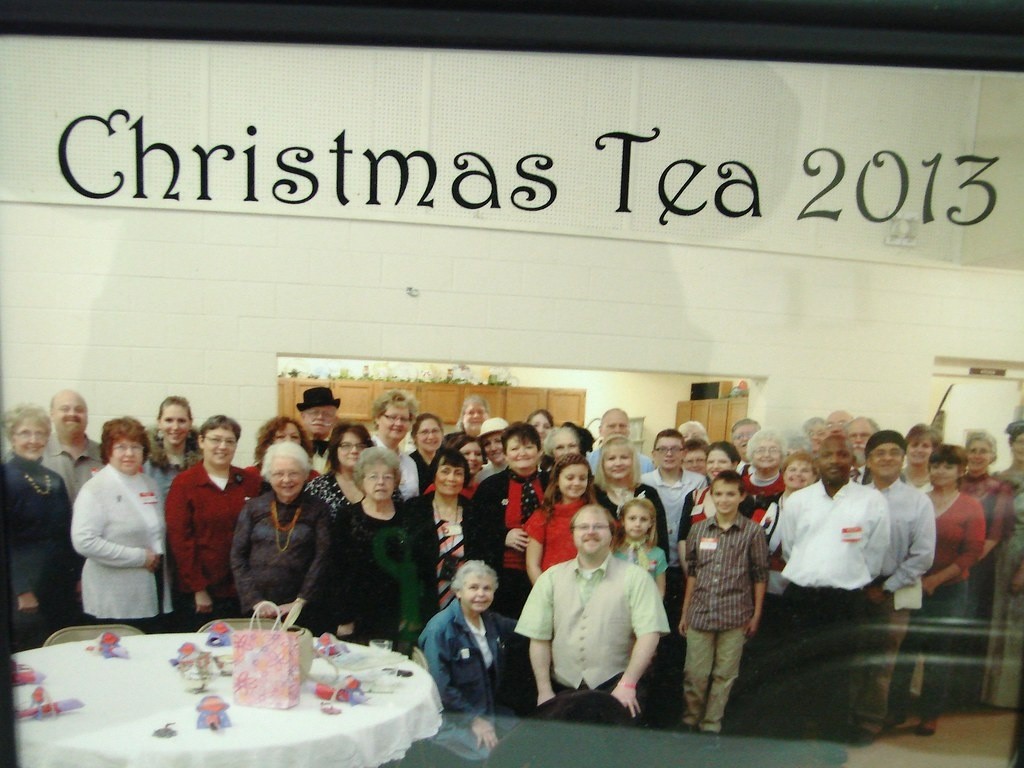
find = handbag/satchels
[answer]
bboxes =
[232,601,301,709]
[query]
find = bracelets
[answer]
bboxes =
[619,683,637,691]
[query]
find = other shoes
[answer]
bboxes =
[698,719,722,733]
[681,711,703,726]
[916,716,938,734]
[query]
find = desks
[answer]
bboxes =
[6,632,445,768]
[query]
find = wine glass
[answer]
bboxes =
[364,639,392,688]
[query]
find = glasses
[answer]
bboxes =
[825,421,846,430]
[654,446,684,455]
[382,413,413,424]
[337,442,368,451]
[205,436,238,447]
[112,443,146,454]
[754,446,782,455]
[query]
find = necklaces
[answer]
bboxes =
[269,497,303,530]
[275,514,293,552]
[20,468,51,494]
[933,490,960,508]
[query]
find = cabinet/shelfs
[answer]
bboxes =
[674,396,756,446]
[278,378,586,439]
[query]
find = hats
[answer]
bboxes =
[476,417,509,437]
[562,421,595,456]
[296,386,340,411]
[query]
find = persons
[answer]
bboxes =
[3,388,1023,760]
